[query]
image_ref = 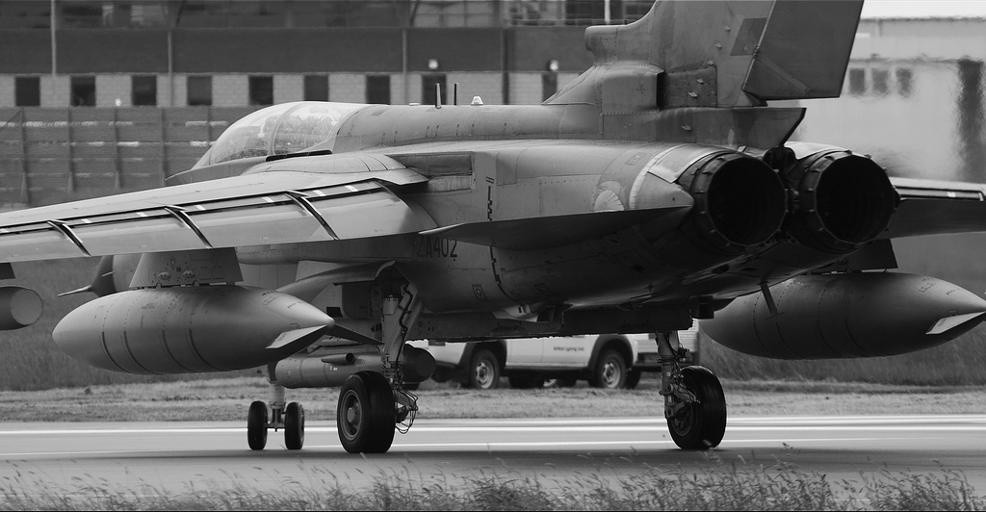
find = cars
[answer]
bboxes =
[401,314,710,391]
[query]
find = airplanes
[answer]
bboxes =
[0,0,986,452]
